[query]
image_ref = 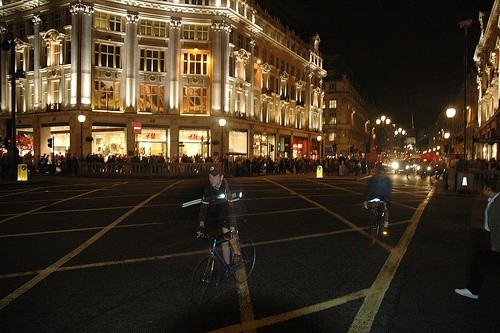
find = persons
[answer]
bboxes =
[364,167,391,229]
[199,165,242,280]
[455,177,500,299]
[0,150,500,193]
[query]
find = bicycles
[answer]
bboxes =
[187,228,256,305]
[361,200,386,246]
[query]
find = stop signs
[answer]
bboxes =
[134,122,142,134]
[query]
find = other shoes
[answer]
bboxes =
[382,227,389,236]
[224,273,232,284]
[455,289,478,299]
[231,254,245,266]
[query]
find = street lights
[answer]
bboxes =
[317,135,322,161]
[77,114,87,156]
[445,104,457,166]
[218,116,226,159]
[456,18,473,170]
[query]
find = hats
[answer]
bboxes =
[209,166,222,174]
